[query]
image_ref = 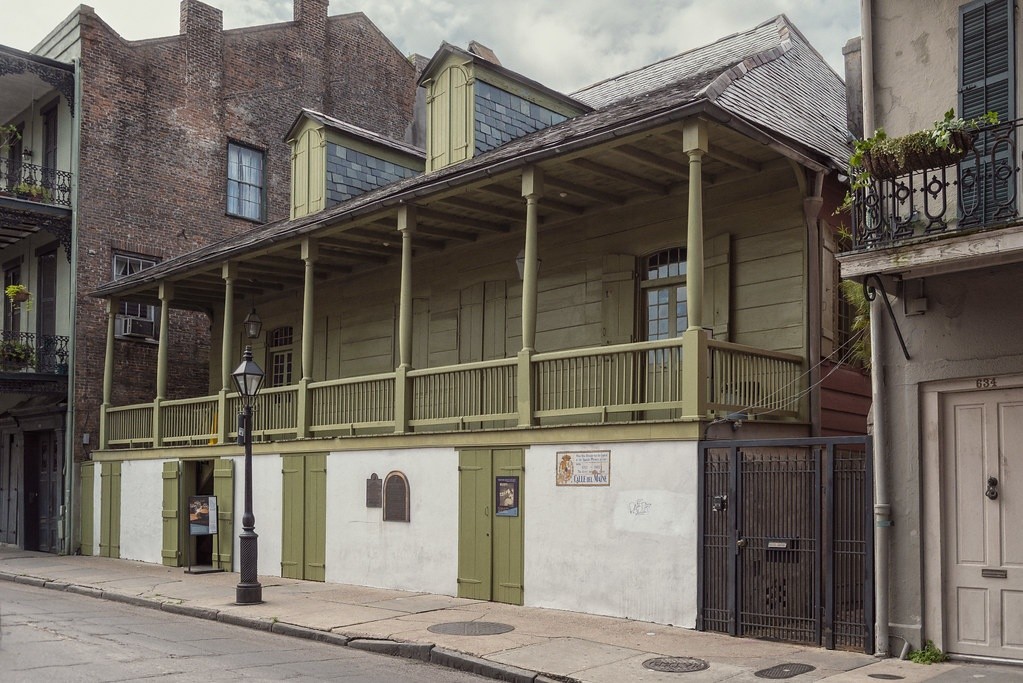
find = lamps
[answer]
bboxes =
[22,146,33,159]
[245,278,263,338]
[515,209,541,282]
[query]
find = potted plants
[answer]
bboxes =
[6,284,35,313]
[839,112,995,210]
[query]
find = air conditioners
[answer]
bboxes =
[123,317,154,338]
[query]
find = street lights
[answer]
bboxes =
[230,344,268,606]
